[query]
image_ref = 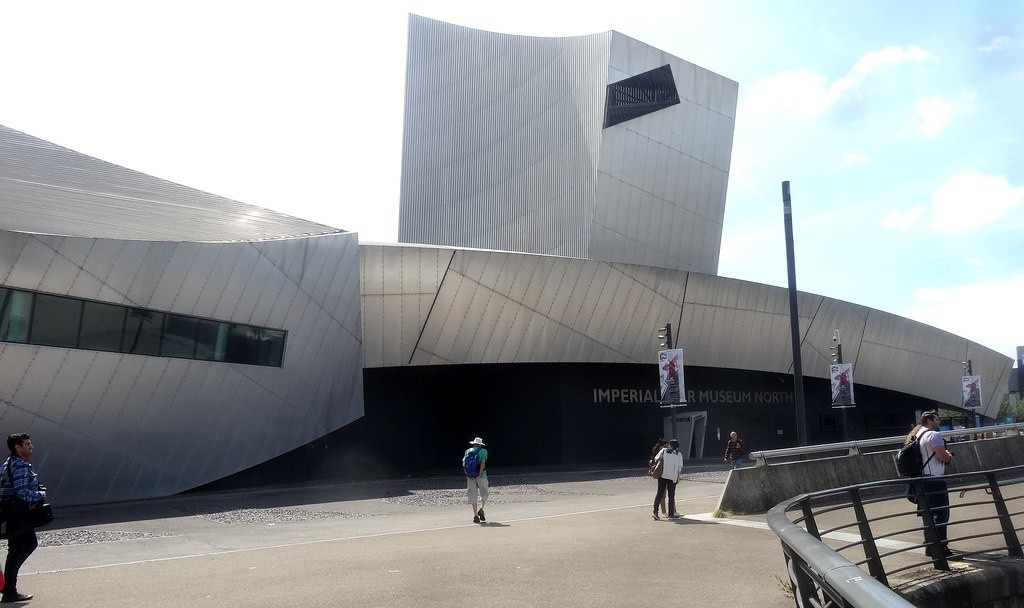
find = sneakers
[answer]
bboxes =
[661,512,668,518]
[478,508,485,521]
[674,511,684,517]
[668,516,680,520]
[473,516,480,523]
[651,514,660,520]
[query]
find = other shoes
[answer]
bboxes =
[2,593,33,603]
[925,549,963,561]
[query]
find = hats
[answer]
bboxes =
[469,437,486,446]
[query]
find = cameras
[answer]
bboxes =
[946,451,954,463]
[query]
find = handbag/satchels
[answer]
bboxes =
[648,450,664,479]
[905,483,918,504]
[29,502,51,522]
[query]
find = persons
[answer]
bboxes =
[462,437,489,523]
[0,433,46,602]
[905,411,964,562]
[724,432,744,470]
[652,438,684,520]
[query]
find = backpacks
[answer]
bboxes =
[896,430,939,478]
[464,448,481,478]
